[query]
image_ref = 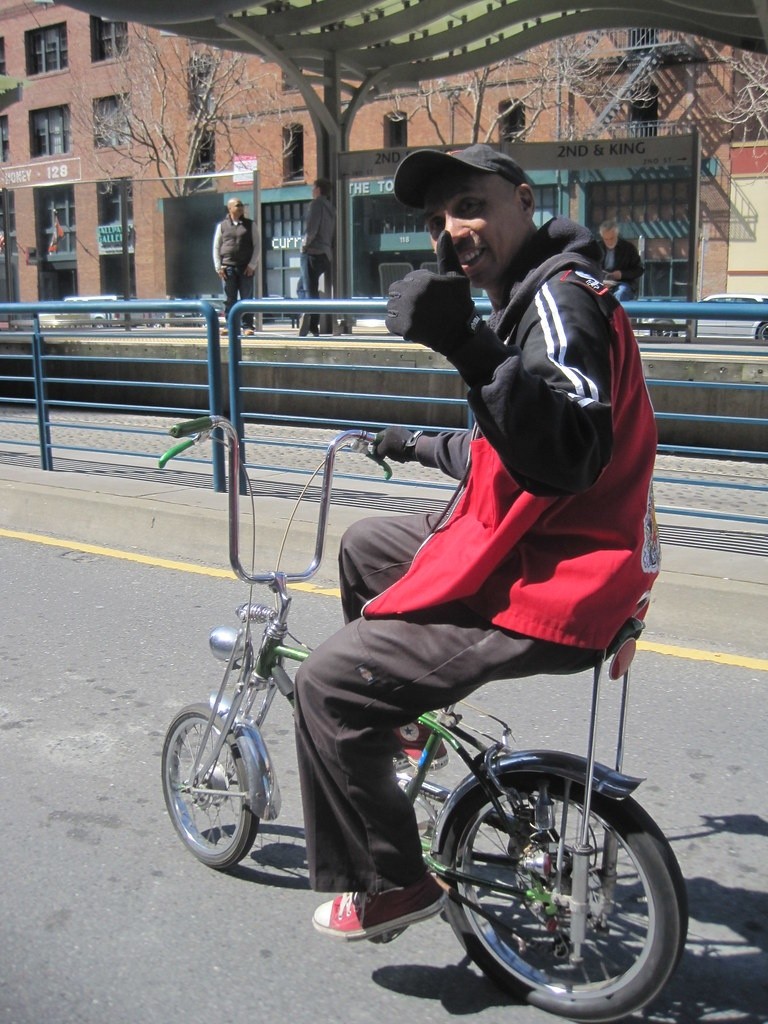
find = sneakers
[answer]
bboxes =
[394,718,451,770]
[311,873,450,936]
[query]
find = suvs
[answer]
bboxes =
[200,299,228,326]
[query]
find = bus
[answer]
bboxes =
[64,294,143,328]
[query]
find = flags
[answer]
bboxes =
[48,216,64,255]
[0,233,6,255]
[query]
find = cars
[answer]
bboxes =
[146,310,204,327]
[637,294,768,343]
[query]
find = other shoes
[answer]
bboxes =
[221,329,255,336]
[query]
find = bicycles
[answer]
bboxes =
[155,418,693,1024]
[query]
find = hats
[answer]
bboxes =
[393,142,530,209]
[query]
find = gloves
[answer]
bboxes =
[383,231,476,353]
[374,428,419,463]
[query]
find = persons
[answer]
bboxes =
[213,199,261,335]
[296,180,337,336]
[290,146,660,940]
[598,222,645,301]
